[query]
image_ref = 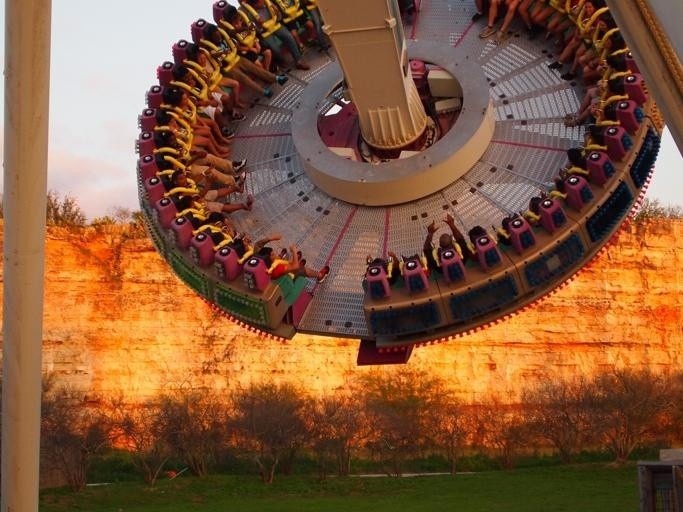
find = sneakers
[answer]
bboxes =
[564,113,584,126]
[263,60,310,97]
[473,13,484,21]
[280,248,330,283]
[549,61,563,68]
[222,112,246,138]
[232,158,253,211]
[479,26,507,45]
[561,72,576,80]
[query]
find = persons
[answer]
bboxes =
[365,213,529,285]
[520,147,592,225]
[153,44,254,217]
[203,1,329,99]
[561,14,630,152]
[199,219,331,284]
[475,1,607,45]
